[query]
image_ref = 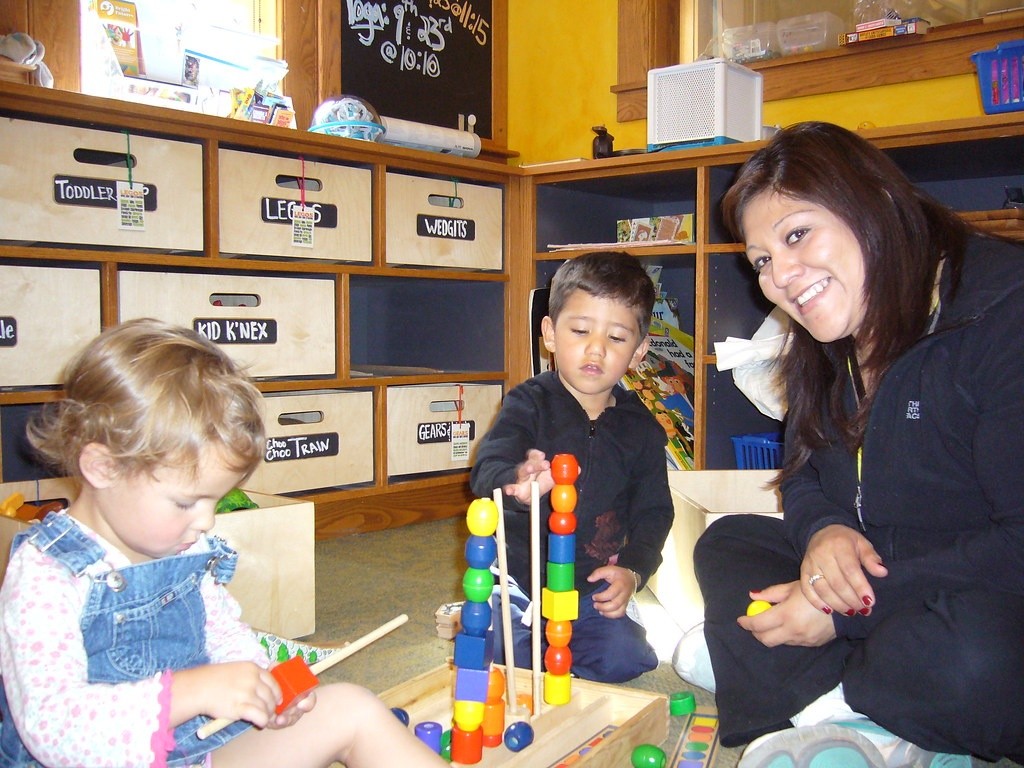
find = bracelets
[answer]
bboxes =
[627,568,638,595]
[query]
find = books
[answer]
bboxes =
[838,17,931,48]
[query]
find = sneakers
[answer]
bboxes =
[675,624,717,696]
[739,717,971,768]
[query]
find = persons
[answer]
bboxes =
[694,122,1023,767]
[0,317,454,768]
[468,251,674,682]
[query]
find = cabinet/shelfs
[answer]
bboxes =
[523,112,1023,470]
[1,81,523,538]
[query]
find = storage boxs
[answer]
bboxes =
[721,21,782,64]
[968,39,1024,115]
[730,429,787,473]
[646,59,765,157]
[776,12,847,57]
[0,486,318,676]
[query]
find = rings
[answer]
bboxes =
[809,574,825,585]
